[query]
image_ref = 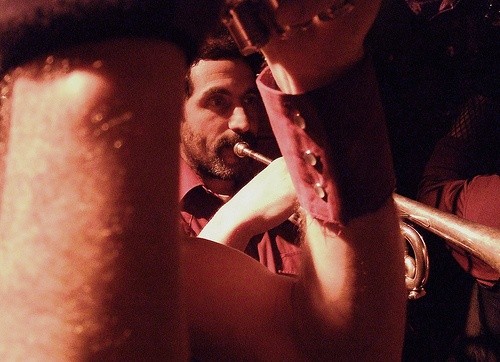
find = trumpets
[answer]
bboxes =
[233,140,500,306]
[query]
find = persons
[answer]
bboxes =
[416,93,500,362]
[174,22,304,281]
[0,0,407,362]
